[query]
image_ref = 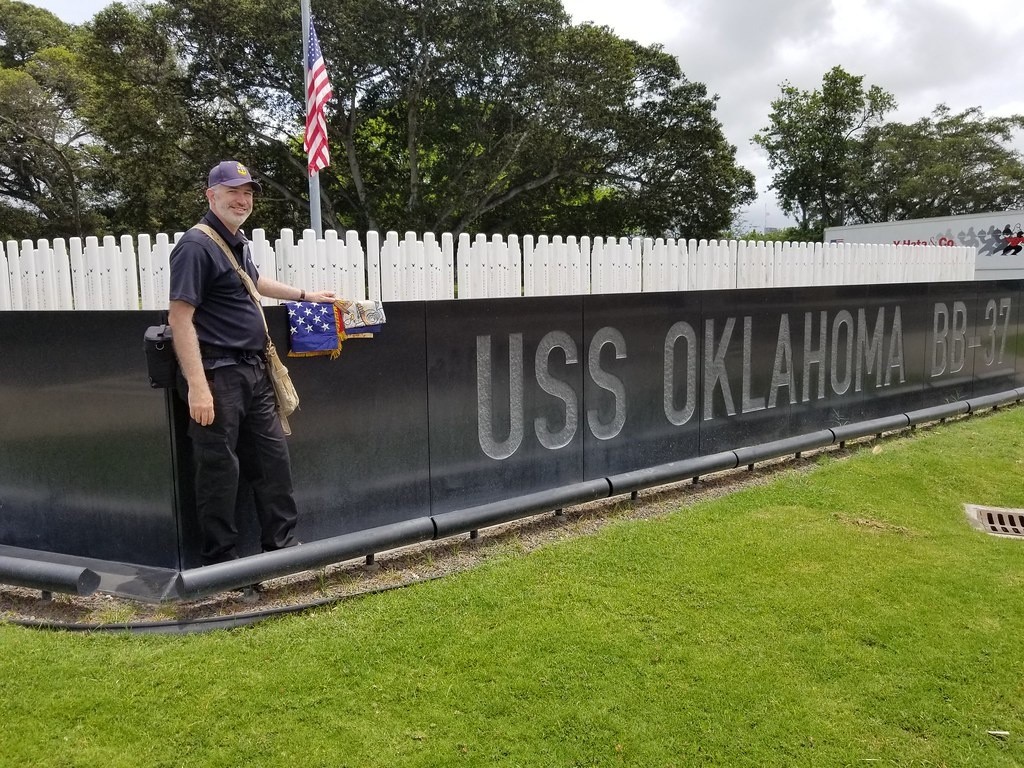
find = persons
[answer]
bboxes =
[168,161,337,592]
[301,0,337,177]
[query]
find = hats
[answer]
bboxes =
[208,161,263,193]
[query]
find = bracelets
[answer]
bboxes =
[299,288,306,301]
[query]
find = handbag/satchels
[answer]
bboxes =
[264,343,299,437]
